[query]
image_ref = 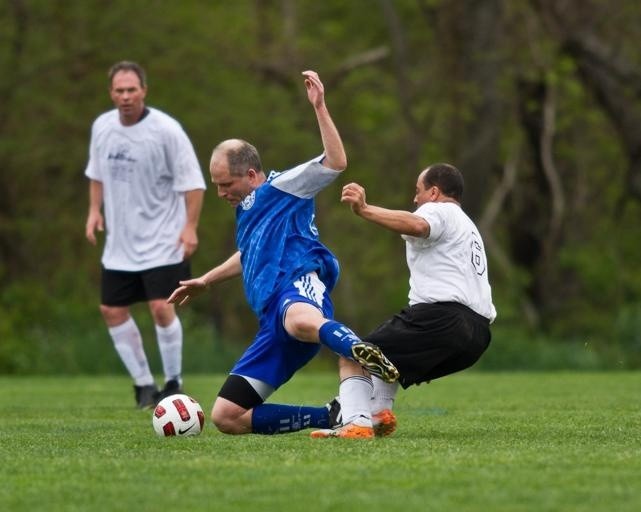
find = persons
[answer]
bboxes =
[84,61,208,409]
[310,162,497,440]
[167,71,400,435]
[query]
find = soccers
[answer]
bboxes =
[152,394,204,436]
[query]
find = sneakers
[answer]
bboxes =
[308,415,376,440]
[324,396,344,431]
[370,408,398,438]
[347,340,401,387]
[130,379,185,412]
